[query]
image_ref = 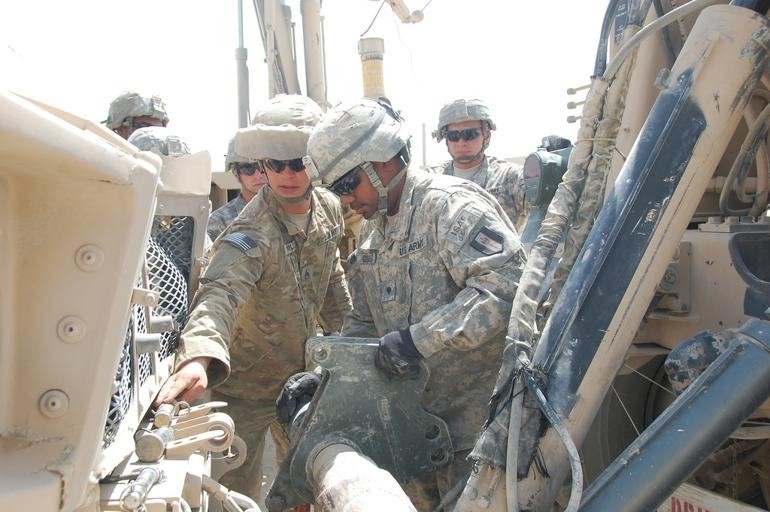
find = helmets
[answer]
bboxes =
[435,98,496,143]
[303,99,412,188]
[107,91,168,129]
[235,95,323,160]
[225,134,258,171]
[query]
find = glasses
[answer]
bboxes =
[230,164,257,176]
[327,168,360,195]
[263,159,305,172]
[442,126,481,142]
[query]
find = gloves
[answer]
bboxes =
[538,136,571,152]
[375,327,425,381]
[275,372,322,424]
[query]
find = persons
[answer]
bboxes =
[303,98,525,512]
[153,94,353,512]
[427,99,529,235]
[206,135,266,244]
[106,91,168,141]
